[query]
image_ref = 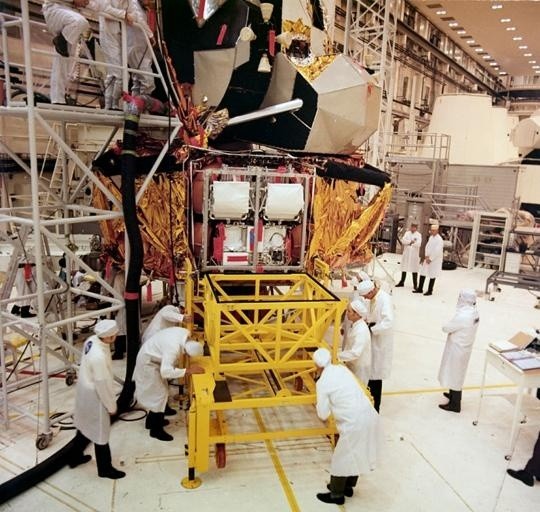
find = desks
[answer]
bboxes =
[469,343,540,462]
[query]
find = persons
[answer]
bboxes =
[506,431,540,486]
[314,224,444,504]
[438,289,479,412]
[42,0,156,113]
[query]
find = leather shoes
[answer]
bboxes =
[326,484,354,497]
[97,468,125,479]
[145,406,177,441]
[395,284,433,296]
[443,392,450,399]
[69,454,91,468]
[507,469,535,487]
[440,405,460,412]
[316,492,345,505]
[11,310,37,318]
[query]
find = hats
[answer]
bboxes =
[358,271,369,281]
[351,300,367,317]
[94,320,119,339]
[184,340,203,358]
[460,288,476,303]
[357,281,374,296]
[312,348,331,368]
[430,224,439,231]
